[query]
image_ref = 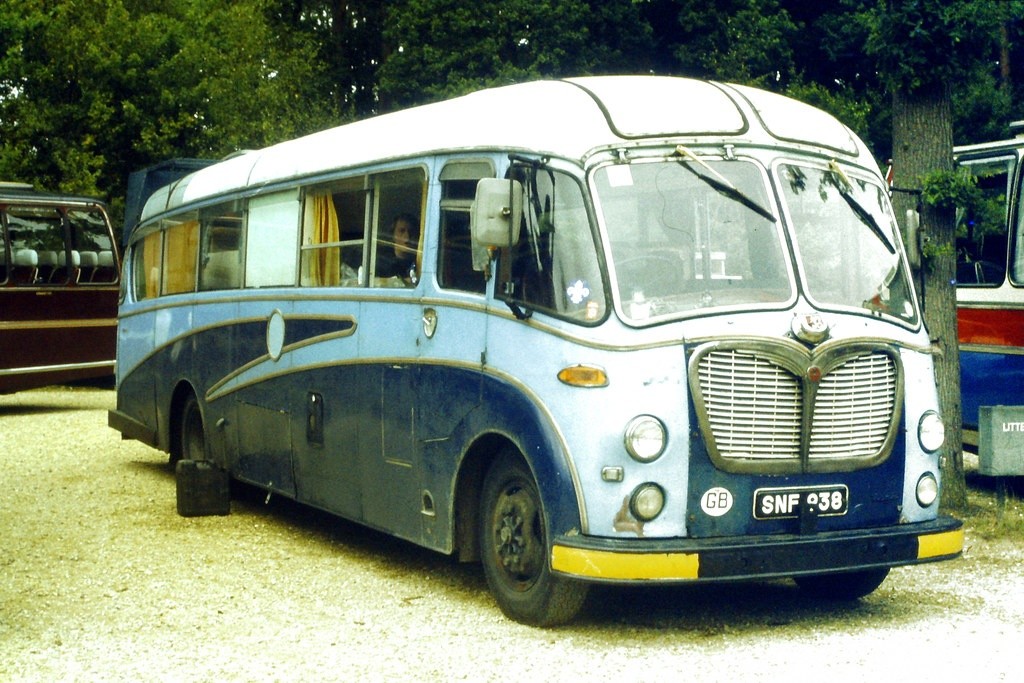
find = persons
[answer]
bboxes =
[359,211,419,288]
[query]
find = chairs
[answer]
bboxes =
[0,250,117,288]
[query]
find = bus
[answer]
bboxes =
[883,134,1024,496]
[0,194,122,397]
[107,73,965,629]
